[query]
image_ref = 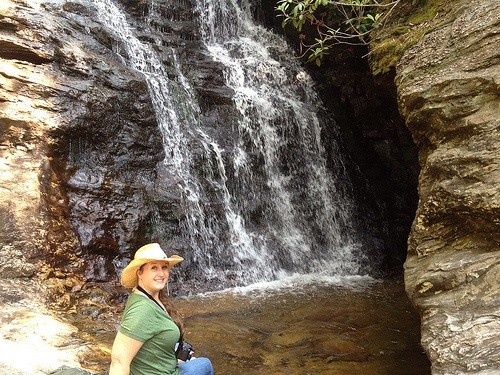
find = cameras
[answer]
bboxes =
[175,342,194,362]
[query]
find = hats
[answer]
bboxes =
[121,243,184,288]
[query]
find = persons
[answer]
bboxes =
[108,243,214,375]
